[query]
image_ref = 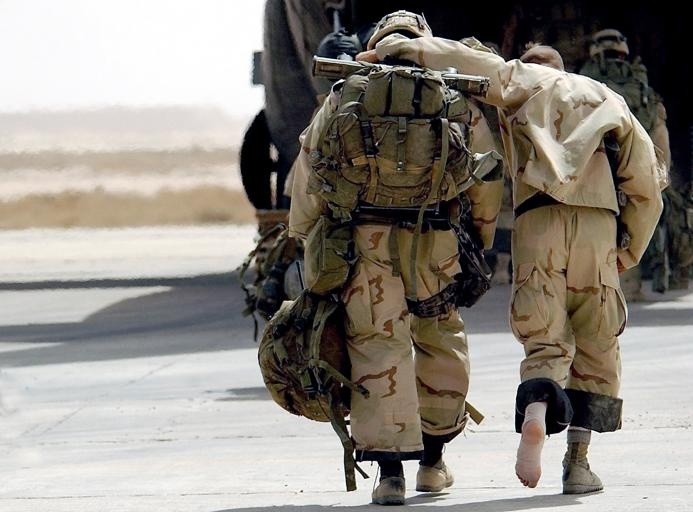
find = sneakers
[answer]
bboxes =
[416,458,453,491]
[372,476,405,504]
[561,463,603,494]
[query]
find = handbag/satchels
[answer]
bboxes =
[304,214,354,295]
[458,232,492,307]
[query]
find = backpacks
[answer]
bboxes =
[306,60,473,219]
[579,56,657,134]
[258,291,345,422]
[238,225,304,313]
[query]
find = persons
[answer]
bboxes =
[575,30,675,303]
[451,1,609,286]
[279,11,506,509]
[310,24,363,124]
[353,35,663,496]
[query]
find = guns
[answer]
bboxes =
[312,55,490,99]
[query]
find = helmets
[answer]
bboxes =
[591,30,631,58]
[365,8,433,52]
